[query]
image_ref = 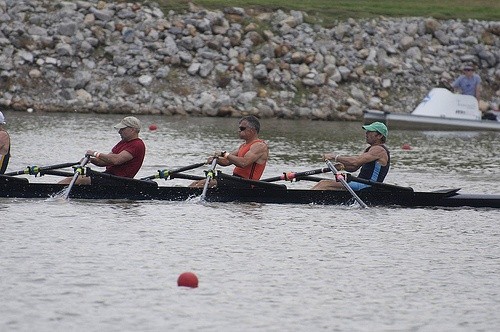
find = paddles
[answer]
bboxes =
[190,156,220,206]
[140,162,206,182]
[260,163,343,183]
[325,156,370,212]
[42,169,74,179]
[2,162,80,177]
[50,155,89,204]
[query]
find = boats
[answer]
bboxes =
[363,80,500,130]
[0,173,500,208]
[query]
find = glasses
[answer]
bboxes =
[239,126,249,131]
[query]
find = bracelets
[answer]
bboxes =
[93,152,102,159]
[334,155,339,164]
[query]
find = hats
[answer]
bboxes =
[463,62,473,69]
[362,121,388,137]
[114,116,141,129]
[0,111,6,124]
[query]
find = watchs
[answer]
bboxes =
[220,151,226,158]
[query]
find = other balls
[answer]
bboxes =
[177,271,199,289]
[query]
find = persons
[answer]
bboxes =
[57,116,145,185]
[311,122,390,191]
[0,111,11,175]
[187,115,268,188]
[449,64,482,111]
